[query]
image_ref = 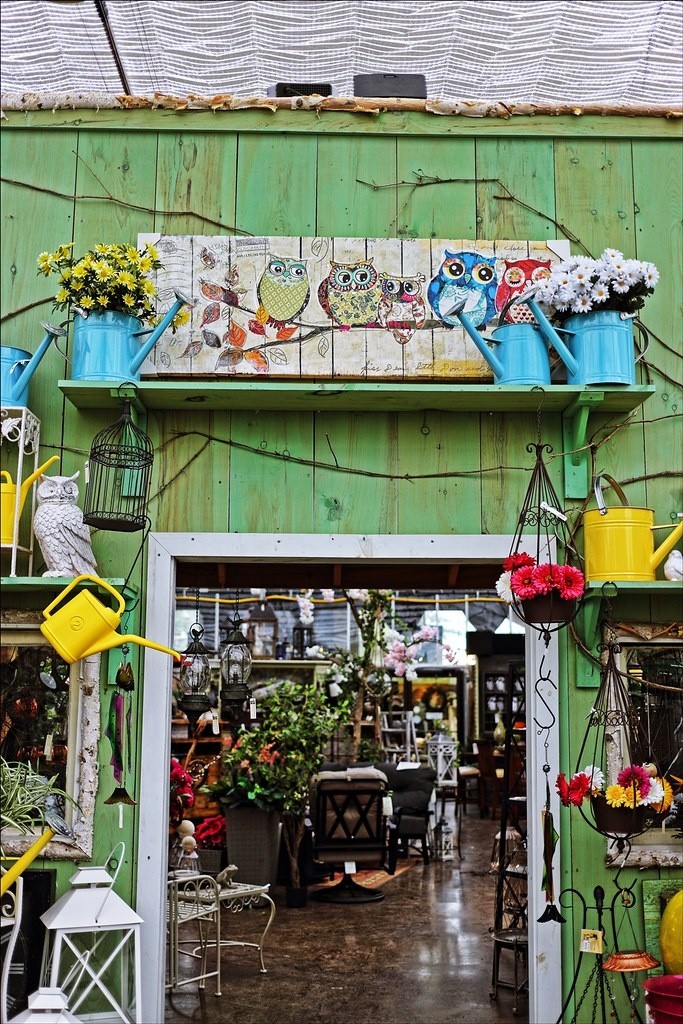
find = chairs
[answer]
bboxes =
[304,778,399,901]
[165,875,222,996]
[430,754,484,819]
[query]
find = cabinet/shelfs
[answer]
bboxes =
[489,796,529,992]
[0,407,41,578]
[466,632,526,819]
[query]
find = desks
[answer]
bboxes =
[172,882,276,988]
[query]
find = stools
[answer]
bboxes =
[489,927,528,1016]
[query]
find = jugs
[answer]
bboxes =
[444,297,551,385]
[1,812,70,898]
[41,575,183,664]
[582,473,683,587]
[0,321,68,411]
[0,452,60,550]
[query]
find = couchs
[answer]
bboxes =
[320,762,438,865]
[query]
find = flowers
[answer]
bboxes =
[170,758,195,822]
[554,763,672,812]
[533,248,658,313]
[495,550,584,602]
[35,242,190,333]
[193,814,228,850]
[381,632,460,680]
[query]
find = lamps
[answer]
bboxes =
[213,589,256,700]
[176,588,213,731]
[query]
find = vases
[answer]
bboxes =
[198,849,222,873]
[521,586,573,623]
[590,795,648,832]
